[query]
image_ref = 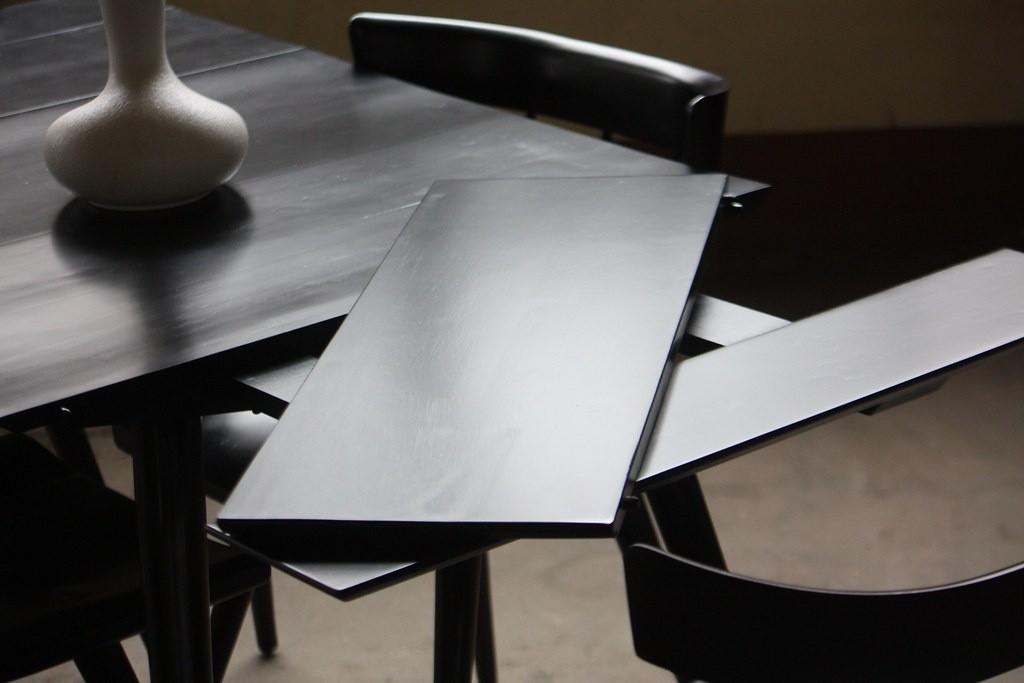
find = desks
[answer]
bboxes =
[0,0,772,683]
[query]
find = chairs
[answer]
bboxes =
[0,13,1024,683]
